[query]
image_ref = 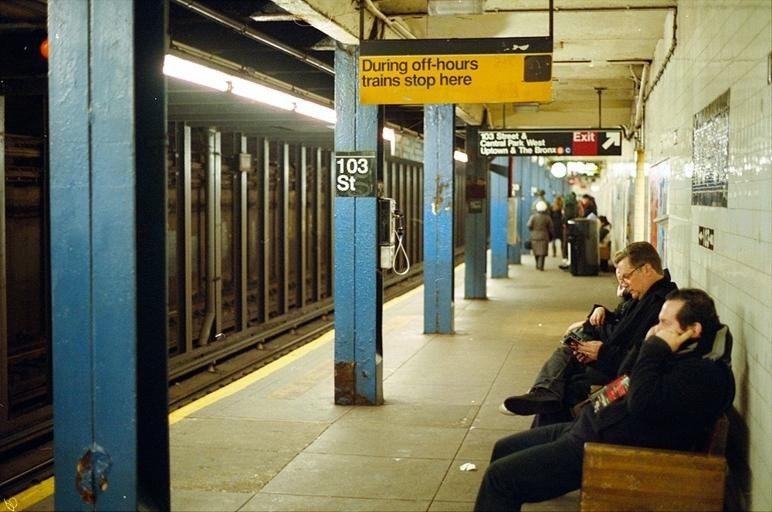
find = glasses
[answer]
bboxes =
[618,263,647,285]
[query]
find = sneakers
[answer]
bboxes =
[503,388,565,417]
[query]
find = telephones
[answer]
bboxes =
[379,197,410,276]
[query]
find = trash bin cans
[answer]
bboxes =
[567,217,600,276]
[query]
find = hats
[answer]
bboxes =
[535,201,548,212]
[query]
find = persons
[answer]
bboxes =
[473,288,724,512]
[560,250,633,344]
[503,241,679,425]
[526,190,597,271]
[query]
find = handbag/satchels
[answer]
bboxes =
[524,240,532,250]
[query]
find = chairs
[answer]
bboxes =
[600,237,611,274]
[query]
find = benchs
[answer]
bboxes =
[580,376,733,511]
